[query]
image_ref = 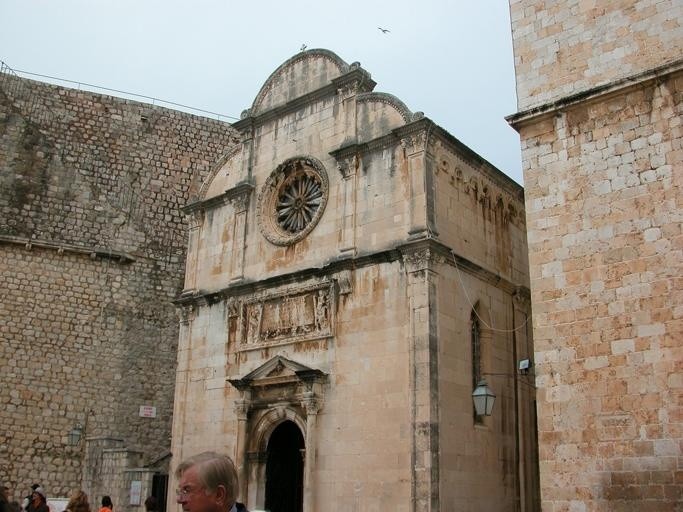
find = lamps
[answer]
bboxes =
[471,373,535,417]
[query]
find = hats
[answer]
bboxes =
[33,488,47,499]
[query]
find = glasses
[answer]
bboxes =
[175,486,193,497]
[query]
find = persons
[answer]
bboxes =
[0,484,114,512]
[173,451,248,512]
